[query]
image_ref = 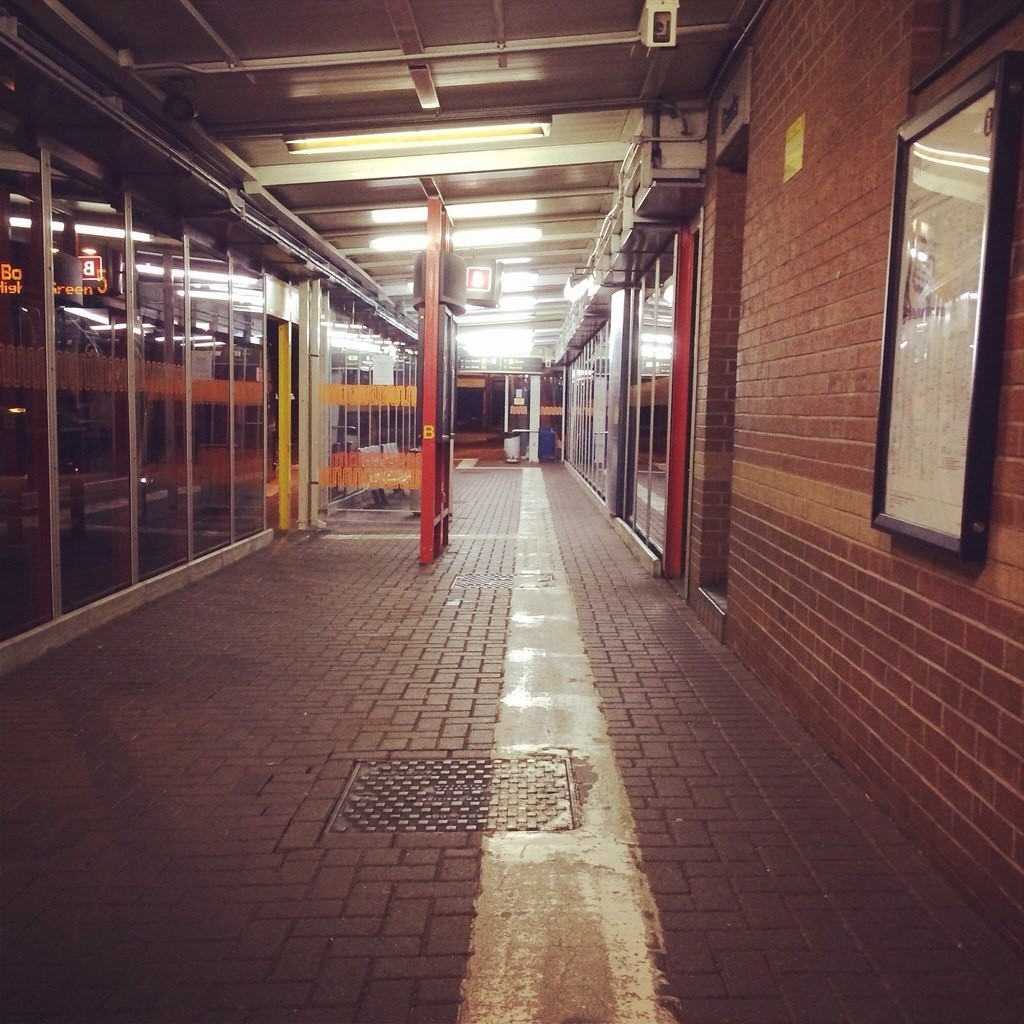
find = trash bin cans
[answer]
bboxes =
[504,432,521,464]
